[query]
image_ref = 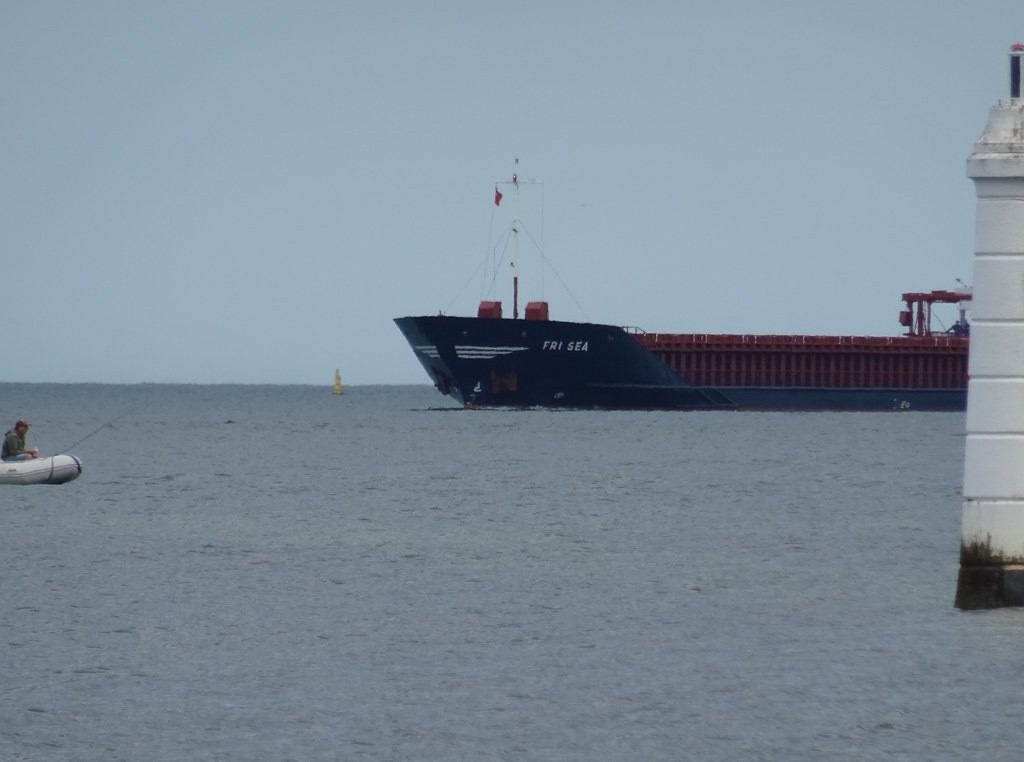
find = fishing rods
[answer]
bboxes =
[52,399,143,457]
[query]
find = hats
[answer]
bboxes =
[16,419,32,428]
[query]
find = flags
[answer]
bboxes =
[495,188,502,206]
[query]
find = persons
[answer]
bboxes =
[961,320,969,336]
[946,321,963,335]
[2,420,39,462]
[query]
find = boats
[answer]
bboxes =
[1,453,84,484]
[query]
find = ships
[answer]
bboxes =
[390,156,970,415]
